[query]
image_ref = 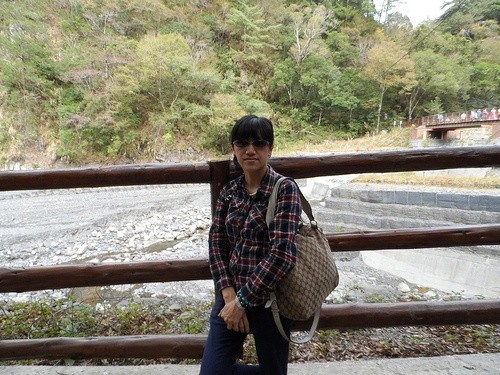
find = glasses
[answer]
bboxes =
[231,139,272,148]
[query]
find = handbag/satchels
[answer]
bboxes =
[266,176,340,321]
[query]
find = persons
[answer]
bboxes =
[197,116,301,375]
